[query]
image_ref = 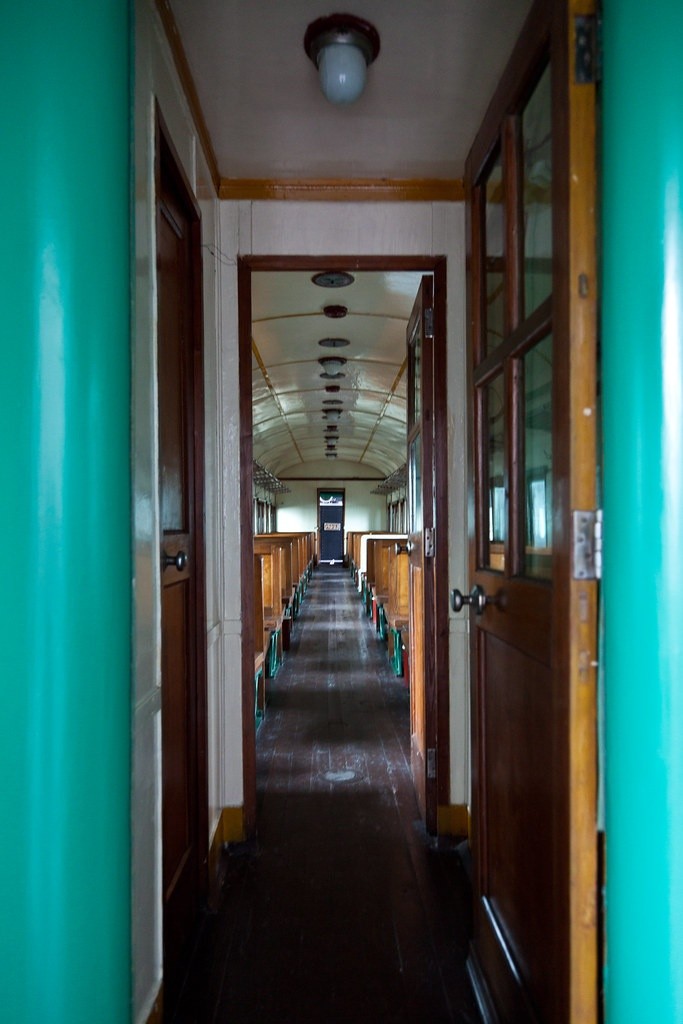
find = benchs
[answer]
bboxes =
[344,531,410,693]
[254,532,317,739]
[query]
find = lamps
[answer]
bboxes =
[304,13,380,106]
[321,409,342,422]
[325,453,337,460]
[318,356,347,376]
[325,436,339,445]
